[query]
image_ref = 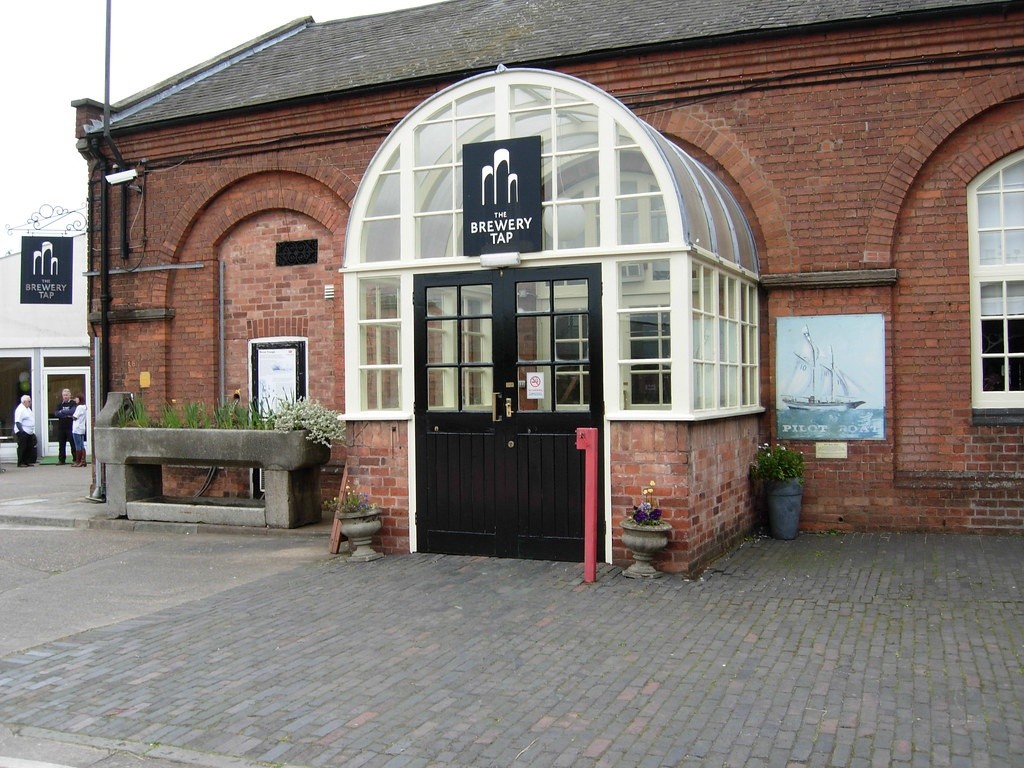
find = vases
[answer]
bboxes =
[766,475,803,540]
[618,520,672,578]
[92,427,331,468]
[336,508,384,563]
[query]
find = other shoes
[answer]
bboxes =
[56,461,65,465]
[18,464,27,467]
[28,464,34,466]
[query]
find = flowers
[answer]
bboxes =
[116,389,346,448]
[623,478,666,527]
[319,486,374,515]
[749,441,807,487]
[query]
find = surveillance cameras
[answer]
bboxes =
[105,169,138,186]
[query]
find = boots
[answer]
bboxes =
[76,449,87,467]
[71,450,82,467]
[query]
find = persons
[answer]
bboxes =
[54,388,77,465]
[71,393,87,467]
[14,395,38,467]
